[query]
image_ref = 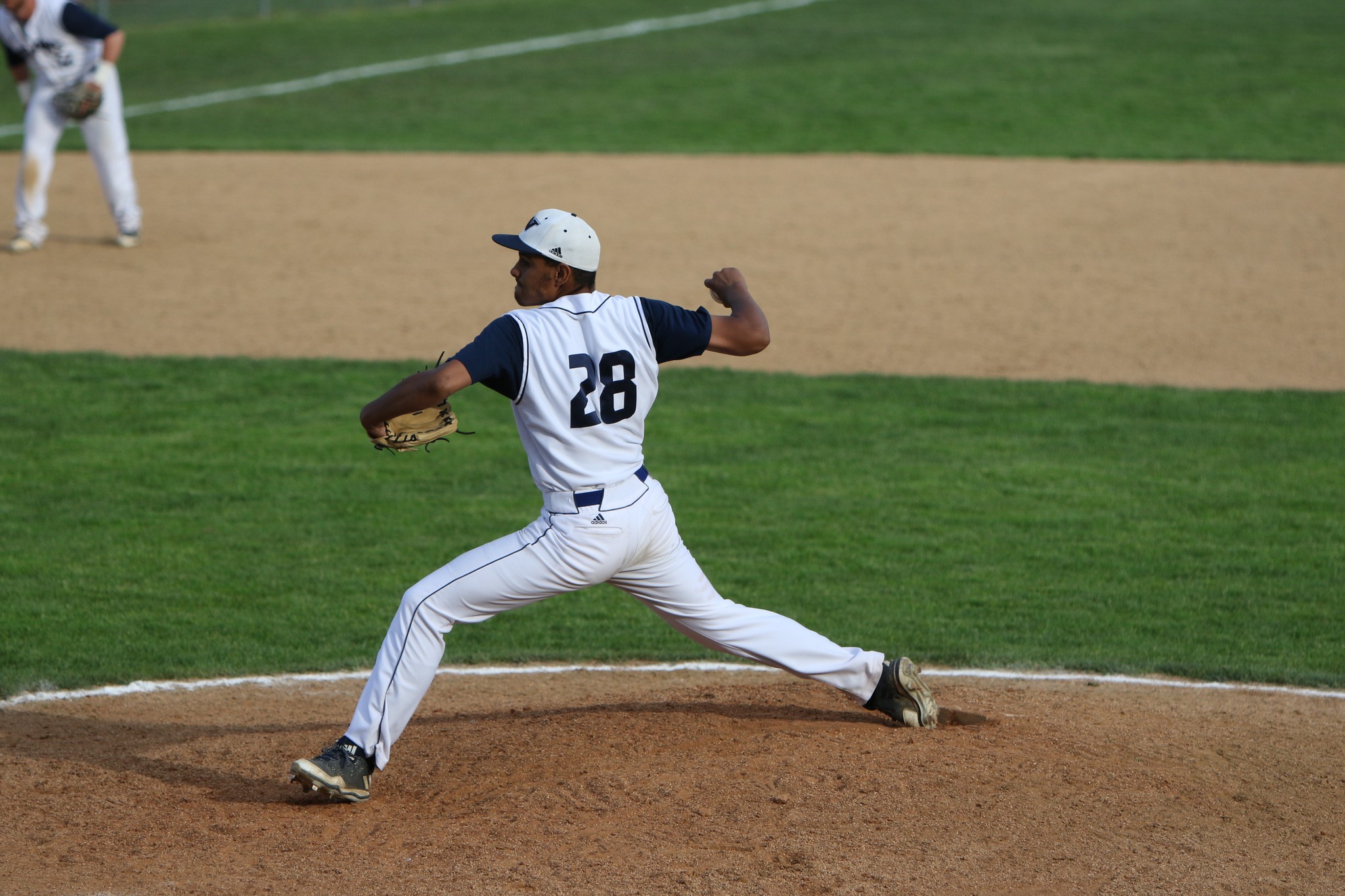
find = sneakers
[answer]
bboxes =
[862,656,939,730]
[289,736,375,803]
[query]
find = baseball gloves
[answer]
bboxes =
[51,82,101,119]
[368,401,459,451]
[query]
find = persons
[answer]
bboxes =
[0,0,142,253]
[291,210,940,802]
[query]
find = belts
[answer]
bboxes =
[575,465,649,507]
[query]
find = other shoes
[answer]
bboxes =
[11,236,33,252]
[116,231,139,248]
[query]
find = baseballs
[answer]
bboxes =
[710,288,723,304]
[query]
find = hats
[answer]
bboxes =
[491,209,600,272]
